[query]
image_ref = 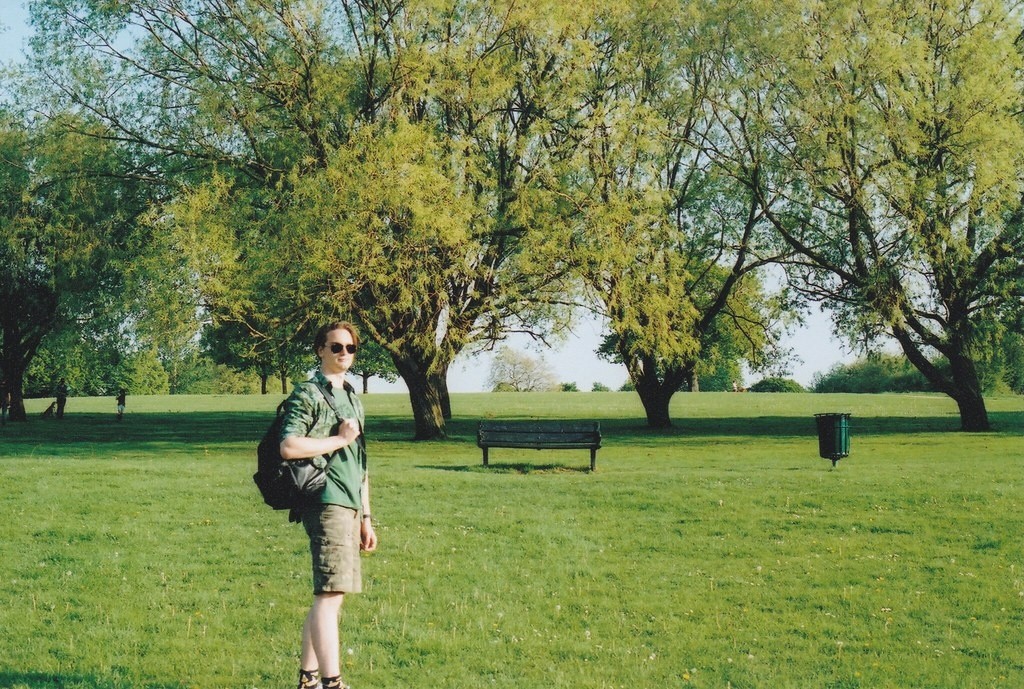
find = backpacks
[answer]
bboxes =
[253,380,367,524]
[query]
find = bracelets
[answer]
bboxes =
[363,513,371,518]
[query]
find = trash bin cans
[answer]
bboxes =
[814,411,849,469]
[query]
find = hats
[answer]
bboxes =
[61,378,65,382]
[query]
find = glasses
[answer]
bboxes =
[324,343,357,354]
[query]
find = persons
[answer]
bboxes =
[276,319,378,689]
[56,378,68,419]
[116,389,125,420]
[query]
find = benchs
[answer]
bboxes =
[477,419,603,471]
